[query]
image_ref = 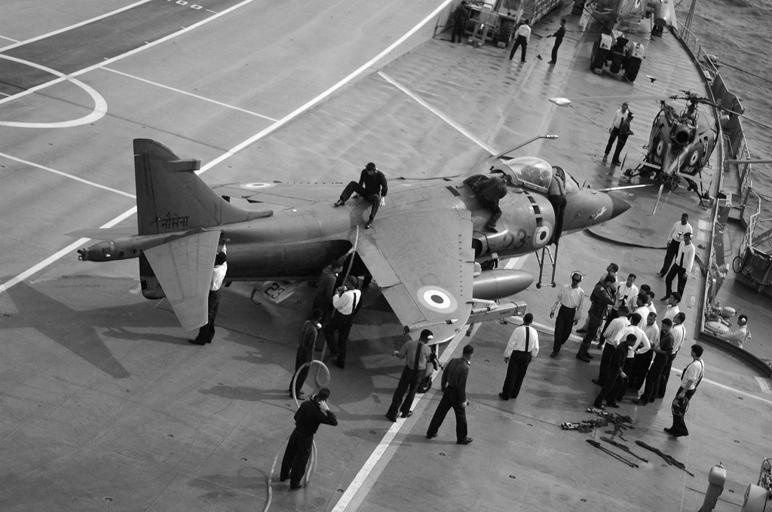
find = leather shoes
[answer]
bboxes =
[288,388,306,401]
[458,438,474,444]
[660,295,670,302]
[663,425,689,436]
[630,396,655,406]
[576,327,586,333]
[365,219,373,229]
[498,391,509,401]
[607,402,620,408]
[188,338,205,346]
[592,378,603,387]
[334,200,345,206]
[385,410,414,422]
[426,432,438,439]
[576,352,593,362]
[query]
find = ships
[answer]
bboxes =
[4,1,772,512]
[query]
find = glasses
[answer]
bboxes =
[571,277,580,282]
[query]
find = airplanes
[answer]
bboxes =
[75,135,633,396]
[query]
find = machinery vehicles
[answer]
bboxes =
[452,0,560,49]
[579,0,679,82]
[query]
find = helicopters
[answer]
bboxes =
[545,72,771,216]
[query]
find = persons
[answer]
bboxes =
[472,174,512,233]
[499,313,539,400]
[604,103,629,161]
[451,0,470,43]
[289,258,361,400]
[547,18,566,63]
[549,212,705,437]
[188,238,230,345]
[612,111,634,166]
[712,314,751,349]
[426,344,474,445]
[510,19,531,62]
[386,329,438,422]
[335,162,388,228]
[280,388,338,490]
